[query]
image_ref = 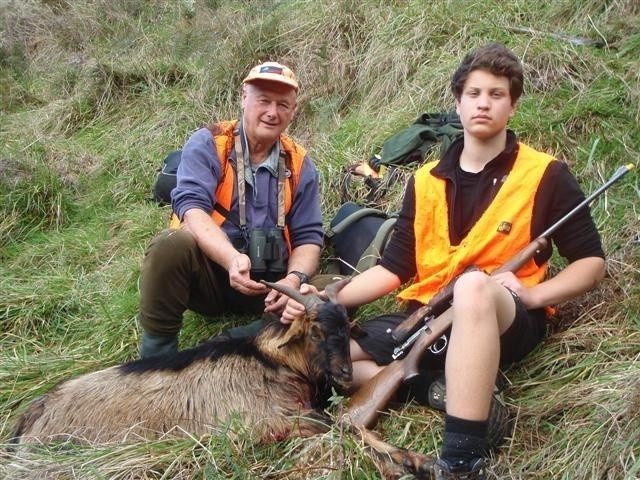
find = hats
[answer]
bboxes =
[242,61,300,97]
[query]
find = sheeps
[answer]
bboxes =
[0,277,459,480]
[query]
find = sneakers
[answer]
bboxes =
[427,367,511,449]
[434,457,485,480]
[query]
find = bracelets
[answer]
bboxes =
[289,271,310,286]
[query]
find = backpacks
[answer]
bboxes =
[324,201,399,277]
[154,150,182,203]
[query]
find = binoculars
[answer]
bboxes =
[250,228,285,273]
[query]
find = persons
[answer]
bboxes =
[278,42,608,480]
[136,60,326,360]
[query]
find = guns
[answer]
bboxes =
[343,162,637,429]
